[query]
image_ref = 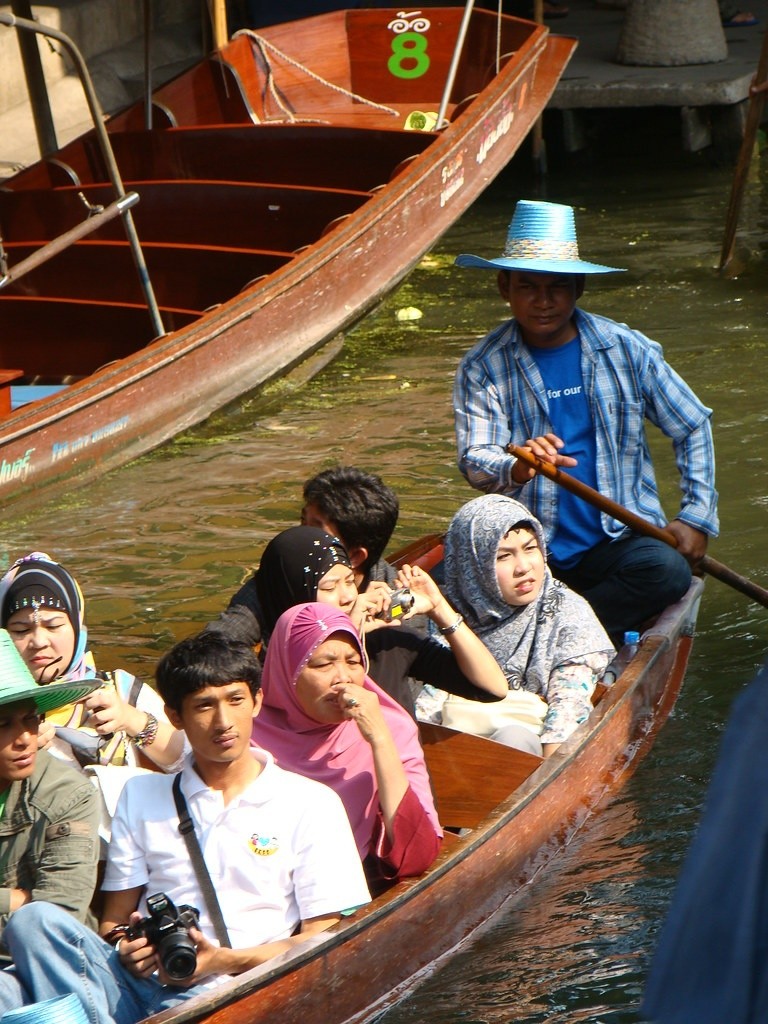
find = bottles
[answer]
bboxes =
[616,631,641,680]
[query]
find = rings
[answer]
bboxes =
[349,699,355,704]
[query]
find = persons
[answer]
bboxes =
[0,467,617,1024]
[453,200,720,634]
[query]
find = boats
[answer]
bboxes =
[0,0,578,516]
[131,529,704,1024]
[639,672,768,1024]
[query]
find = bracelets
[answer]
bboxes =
[438,613,463,635]
[132,712,158,749]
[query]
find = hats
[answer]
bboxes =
[1,628,103,712]
[453,198,630,276]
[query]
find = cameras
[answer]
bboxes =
[126,892,204,978]
[381,587,414,624]
